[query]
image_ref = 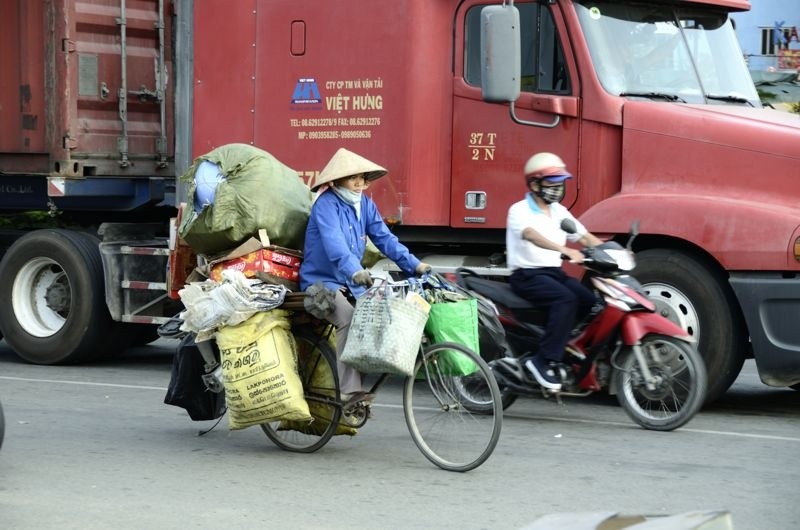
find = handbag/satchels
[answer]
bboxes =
[429,272,508,363]
[403,281,481,381]
[343,277,431,377]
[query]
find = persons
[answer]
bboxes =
[505,151,604,389]
[300,149,434,418]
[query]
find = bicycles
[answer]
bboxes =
[258,287,503,472]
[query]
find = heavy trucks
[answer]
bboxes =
[0,1,800,409]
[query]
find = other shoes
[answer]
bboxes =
[343,391,376,411]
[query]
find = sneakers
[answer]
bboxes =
[525,358,562,389]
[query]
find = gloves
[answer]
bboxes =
[415,263,433,275]
[352,272,372,287]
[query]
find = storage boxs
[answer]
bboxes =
[209,250,301,284]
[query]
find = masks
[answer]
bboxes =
[535,181,564,203]
[334,186,362,203]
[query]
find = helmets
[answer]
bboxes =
[523,153,571,188]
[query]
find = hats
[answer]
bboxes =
[543,176,572,183]
[310,148,388,192]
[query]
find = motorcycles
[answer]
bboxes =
[459,219,707,432]
